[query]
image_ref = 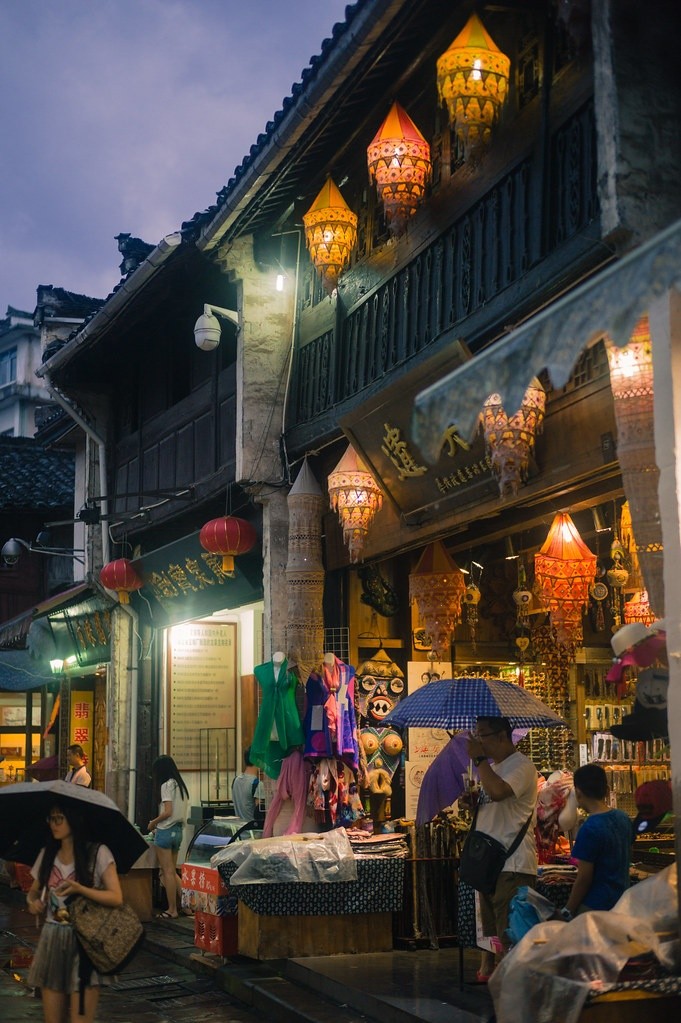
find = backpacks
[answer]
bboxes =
[63,842,147,1015]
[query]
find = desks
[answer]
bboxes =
[218,855,408,960]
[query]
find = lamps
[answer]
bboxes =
[35,508,151,548]
[325,442,380,565]
[608,309,663,626]
[474,366,545,492]
[78,486,195,527]
[437,17,510,142]
[406,539,465,661]
[301,176,356,292]
[366,103,430,226]
[534,511,596,639]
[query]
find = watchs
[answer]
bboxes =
[474,756,487,767]
[561,907,573,922]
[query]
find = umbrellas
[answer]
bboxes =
[25,753,60,782]
[381,678,563,791]
[0,780,149,874]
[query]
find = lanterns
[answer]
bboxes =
[200,510,258,572]
[100,556,145,605]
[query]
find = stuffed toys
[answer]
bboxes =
[536,765,579,832]
[609,668,670,745]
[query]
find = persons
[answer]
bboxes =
[64,745,92,788]
[454,863,497,983]
[230,746,263,819]
[546,763,636,924]
[146,756,193,919]
[415,727,484,827]
[26,803,123,1023]
[448,716,540,956]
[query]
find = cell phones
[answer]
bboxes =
[55,882,70,894]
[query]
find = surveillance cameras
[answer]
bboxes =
[1,540,23,565]
[194,314,221,351]
[36,531,51,547]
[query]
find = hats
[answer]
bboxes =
[610,668,669,742]
[633,780,673,835]
[605,622,666,682]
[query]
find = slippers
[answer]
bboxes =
[155,911,179,919]
[178,909,194,916]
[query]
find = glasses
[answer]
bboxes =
[46,813,64,825]
[473,730,502,739]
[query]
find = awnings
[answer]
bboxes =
[1,579,106,651]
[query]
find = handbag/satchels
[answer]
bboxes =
[457,831,508,896]
